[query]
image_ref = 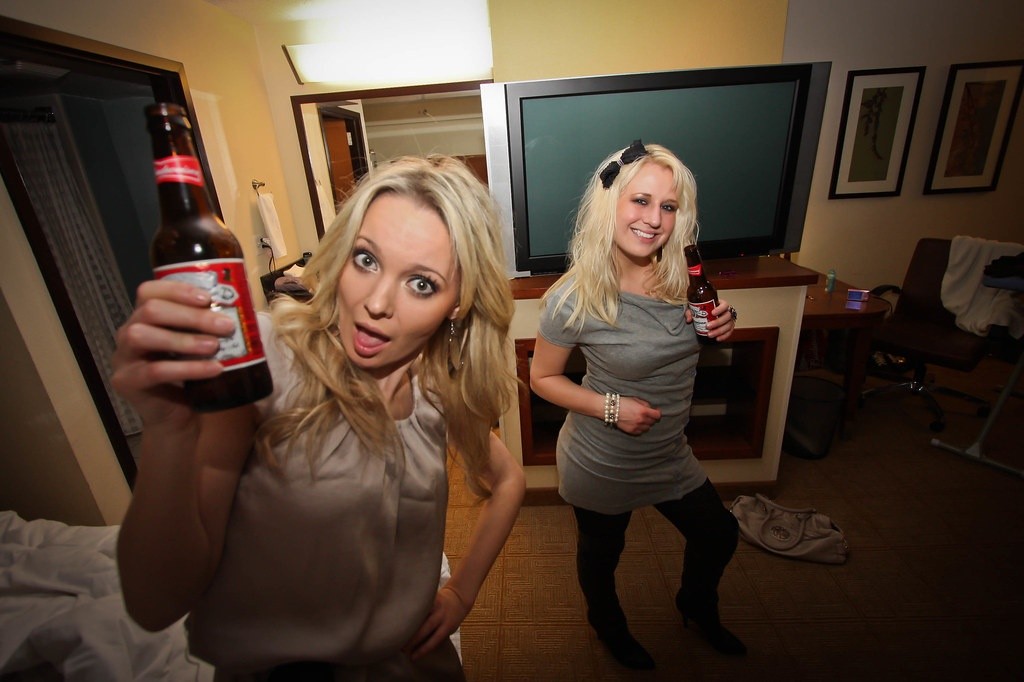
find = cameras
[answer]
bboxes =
[847,289,870,301]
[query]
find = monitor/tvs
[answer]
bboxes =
[479,61,832,274]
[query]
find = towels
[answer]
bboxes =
[939,234,1024,340]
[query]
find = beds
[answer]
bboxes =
[0,509,463,682]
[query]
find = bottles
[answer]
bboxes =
[825,269,835,293]
[139,101,274,413]
[683,244,724,346]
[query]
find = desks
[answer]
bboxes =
[799,264,892,442]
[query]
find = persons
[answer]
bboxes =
[529,141,748,668]
[110,149,528,681]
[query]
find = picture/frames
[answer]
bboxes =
[922,58,1024,195]
[828,65,928,200]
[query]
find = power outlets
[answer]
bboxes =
[253,232,269,256]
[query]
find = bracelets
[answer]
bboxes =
[605,392,620,425]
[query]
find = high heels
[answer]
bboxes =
[676,592,746,656]
[588,607,655,669]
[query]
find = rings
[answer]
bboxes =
[728,304,737,319]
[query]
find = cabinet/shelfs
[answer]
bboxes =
[498,258,818,507]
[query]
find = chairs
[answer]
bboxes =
[875,236,1024,434]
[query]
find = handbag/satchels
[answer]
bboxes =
[730,491,849,564]
